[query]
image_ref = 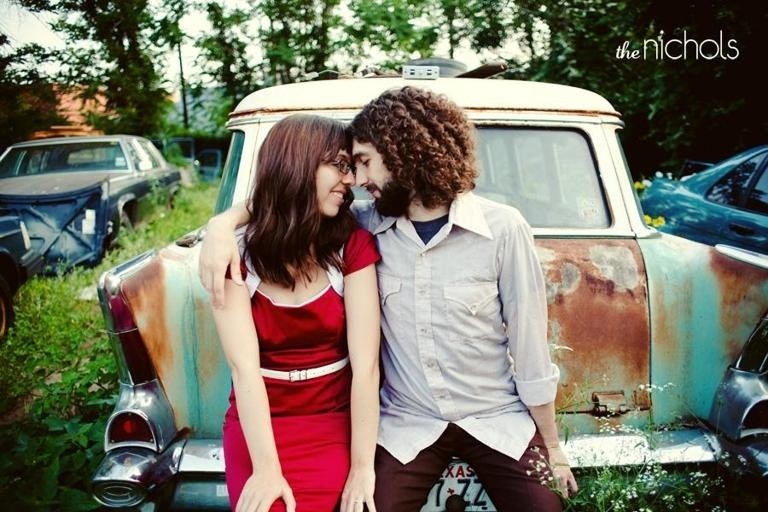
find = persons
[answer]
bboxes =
[199,86,579,512]
[207,114,381,512]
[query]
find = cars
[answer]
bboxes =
[0,133,183,343]
[93,77,766,512]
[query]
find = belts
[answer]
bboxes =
[261,355,350,384]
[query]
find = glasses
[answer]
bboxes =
[321,158,357,175]
